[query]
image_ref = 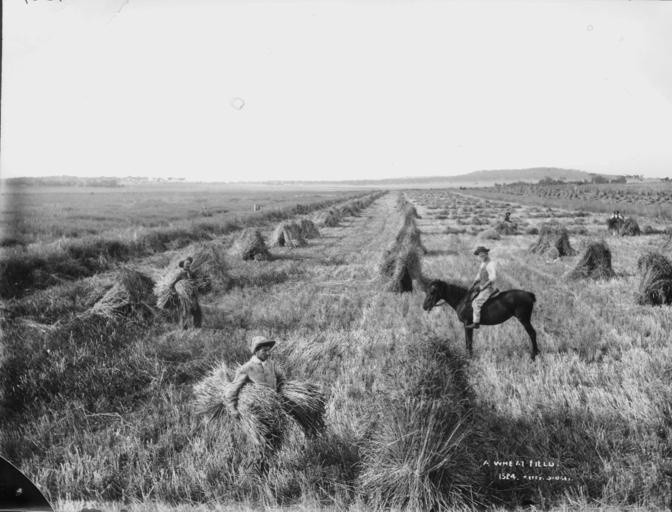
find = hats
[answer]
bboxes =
[251,335,276,356]
[473,247,489,256]
[179,257,192,268]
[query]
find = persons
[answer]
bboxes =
[169,257,202,328]
[465,247,496,329]
[504,212,512,235]
[226,336,286,474]
[610,210,623,236]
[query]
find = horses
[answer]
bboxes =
[421,278,539,361]
